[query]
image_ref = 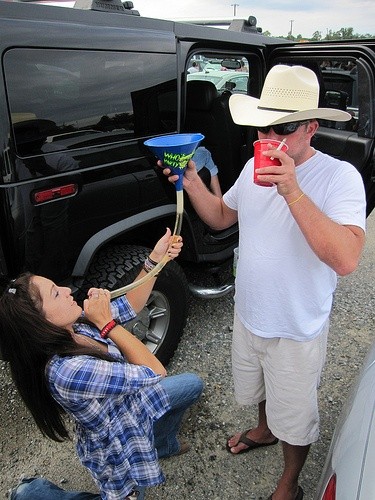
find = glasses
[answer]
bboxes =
[256,119,311,135]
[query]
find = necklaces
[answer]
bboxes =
[75,334,103,352]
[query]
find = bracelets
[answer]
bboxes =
[143,255,160,274]
[100,317,123,339]
[287,193,305,208]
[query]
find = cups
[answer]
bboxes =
[253,139,288,187]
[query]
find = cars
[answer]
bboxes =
[33,63,82,101]
[185,70,249,94]
[189,59,249,71]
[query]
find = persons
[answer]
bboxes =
[189,144,223,201]
[0,118,84,290]
[184,58,354,76]
[0,226,206,500]
[156,64,367,500]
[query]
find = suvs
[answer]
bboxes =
[1,0,375,370]
[321,70,361,132]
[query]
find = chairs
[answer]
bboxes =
[185,78,247,196]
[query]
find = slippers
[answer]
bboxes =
[267,485,303,500]
[226,428,279,454]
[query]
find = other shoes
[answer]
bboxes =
[10,477,36,500]
[174,440,191,455]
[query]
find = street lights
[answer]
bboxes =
[289,20,295,36]
[230,4,240,18]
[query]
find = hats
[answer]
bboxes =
[228,64,352,127]
